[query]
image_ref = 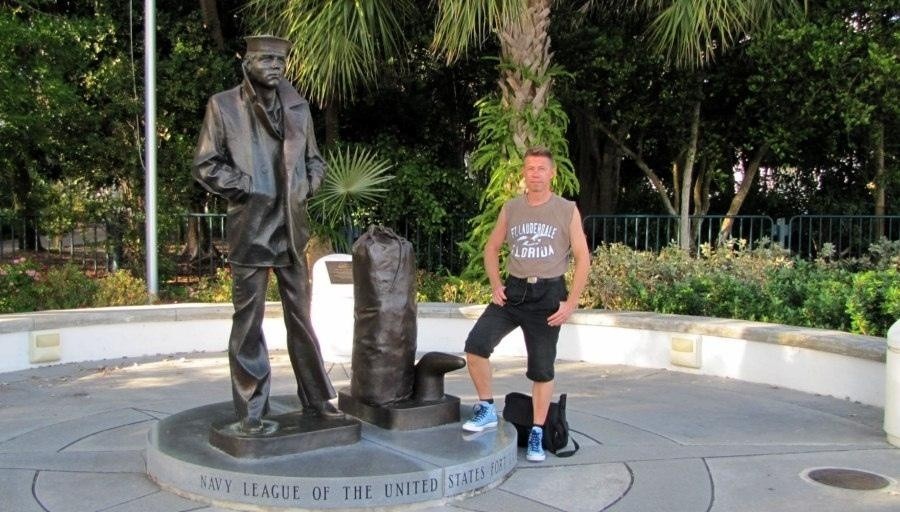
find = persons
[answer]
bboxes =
[187,33,344,440]
[460,148,591,464]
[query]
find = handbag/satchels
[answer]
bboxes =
[503,391,579,458]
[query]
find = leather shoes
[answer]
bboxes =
[302,405,346,418]
[239,418,263,433]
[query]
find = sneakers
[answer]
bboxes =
[460,402,498,431]
[525,426,546,462]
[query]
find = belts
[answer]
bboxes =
[508,273,564,285]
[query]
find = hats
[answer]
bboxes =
[244,35,291,57]
